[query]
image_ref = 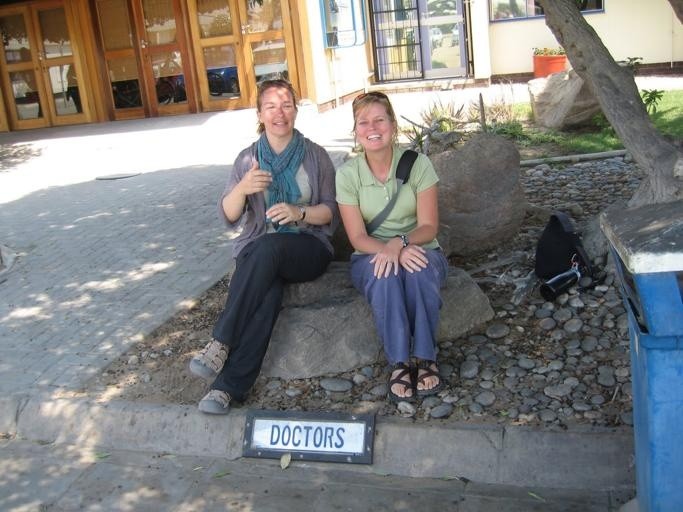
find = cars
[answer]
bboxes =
[451,23,460,47]
[249,42,289,87]
[176,46,240,94]
[430,27,443,48]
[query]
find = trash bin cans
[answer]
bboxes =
[597,200,682,512]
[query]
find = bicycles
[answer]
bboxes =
[150,62,175,106]
[175,71,226,104]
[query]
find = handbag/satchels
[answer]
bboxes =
[535,215,591,279]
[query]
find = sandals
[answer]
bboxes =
[416,358,444,397]
[389,363,416,402]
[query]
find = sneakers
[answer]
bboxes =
[199,389,233,414]
[189,337,231,378]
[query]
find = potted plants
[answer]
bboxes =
[532,46,568,76]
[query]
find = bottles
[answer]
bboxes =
[540,266,589,302]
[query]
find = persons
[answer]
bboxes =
[188,78,340,416]
[335,90,449,402]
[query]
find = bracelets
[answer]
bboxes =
[298,205,306,219]
[401,233,409,247]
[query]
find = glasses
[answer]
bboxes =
[353,92,390,120]
